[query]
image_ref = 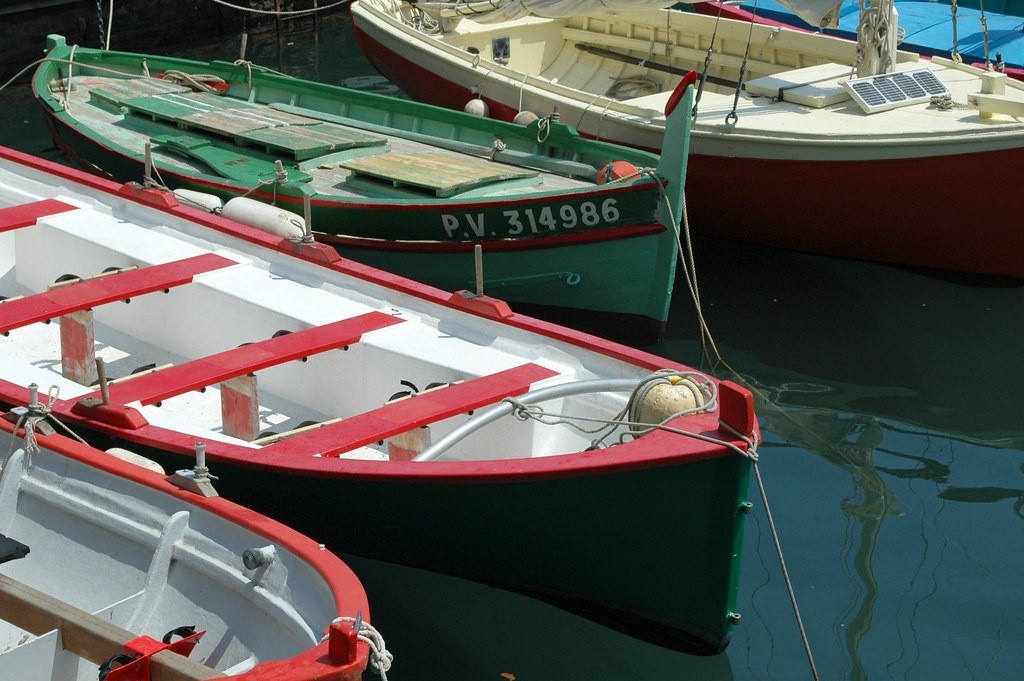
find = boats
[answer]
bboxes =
[30,31,697,323]
[683,0,1024,82]
[0,147,760,659]
[0,404,371,680]
[346,0,1024,289]
